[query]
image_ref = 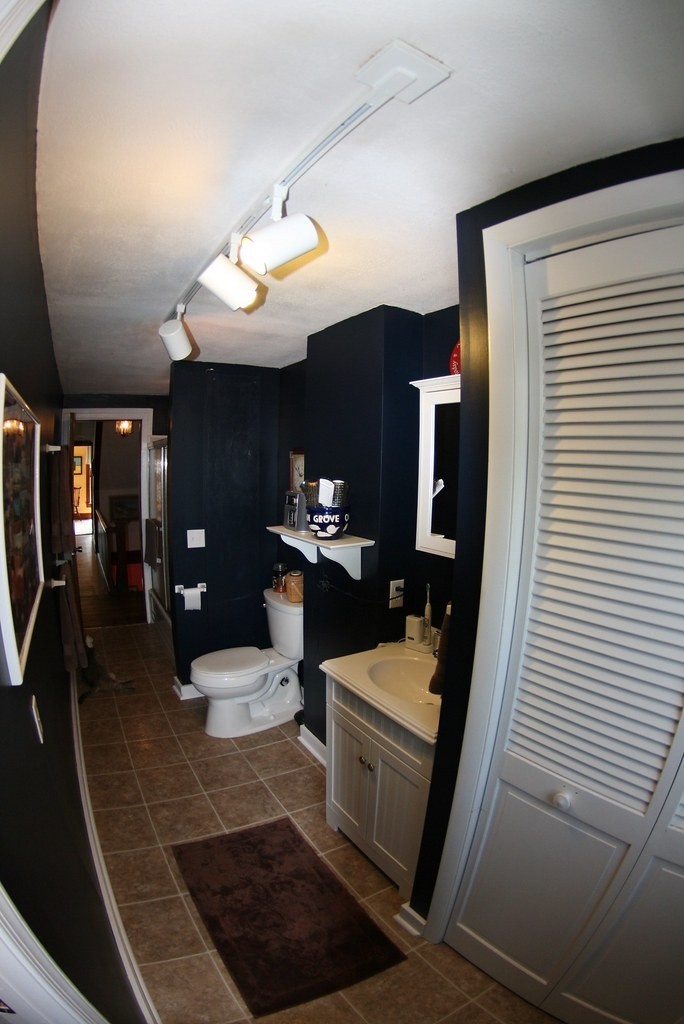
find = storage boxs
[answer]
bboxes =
[112,562,143,592]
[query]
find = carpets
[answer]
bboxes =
[171,817,409,1019]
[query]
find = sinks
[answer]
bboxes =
[364,654,443,707]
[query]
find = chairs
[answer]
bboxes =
[72,487,82,521]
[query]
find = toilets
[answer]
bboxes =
[189,587,305,739]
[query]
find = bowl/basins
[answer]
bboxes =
[305,505,353,540]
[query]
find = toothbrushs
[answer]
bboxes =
[423,583,432,647]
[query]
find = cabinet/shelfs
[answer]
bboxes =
[393,138,684,1024]
[326,677,438,900]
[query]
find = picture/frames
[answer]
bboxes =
[109,494,140,522]
[0,371,44,687]
[74,456,82,475]
[289,448,304,492]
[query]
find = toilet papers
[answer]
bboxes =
[183,588,201,611]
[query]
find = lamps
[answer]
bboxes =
[116,420,133,440]
[196,230,259,310]
[159,302,192,362]
[238,187,318,274]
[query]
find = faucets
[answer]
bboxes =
[432,648,440,659]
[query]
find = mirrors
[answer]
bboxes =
[409,374,461,559]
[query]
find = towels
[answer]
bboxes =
[428,600,452,695]
